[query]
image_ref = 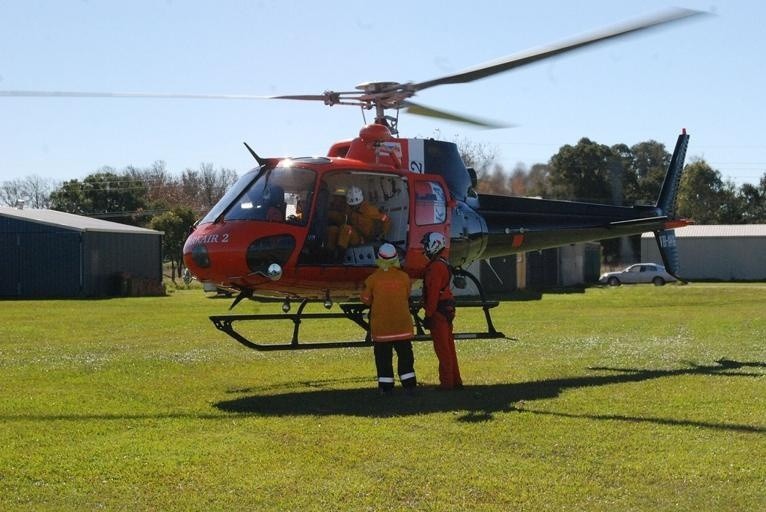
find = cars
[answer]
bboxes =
[599,261,676,288]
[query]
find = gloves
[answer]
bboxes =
[422,314,432,331]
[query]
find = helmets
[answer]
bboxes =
[377,243,398,261]
[346,186,364,206]
[420,231,445,259]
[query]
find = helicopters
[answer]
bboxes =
[0,9,713,350]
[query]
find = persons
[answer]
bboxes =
[360,243,421,393]
[326,186,391,263]
[323,186,347,225]
[293,190,309,219]
[418,230,465,392]
